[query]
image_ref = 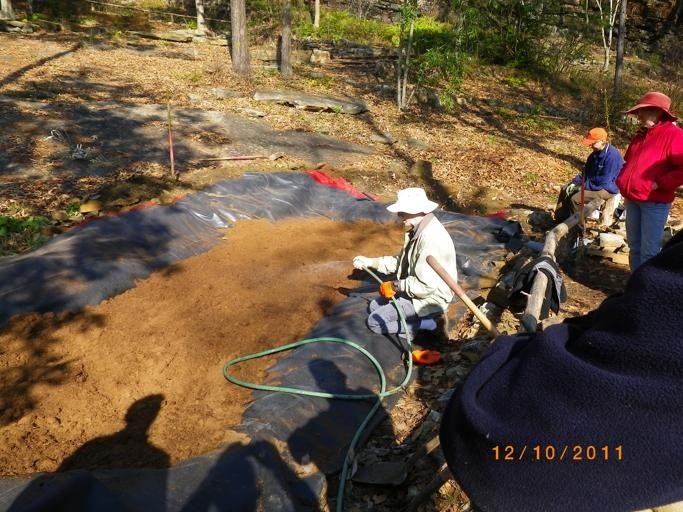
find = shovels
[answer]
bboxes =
[352,434,441,486]
[575,169,587,264]
[199,151,286,161]
[167,103,179,182]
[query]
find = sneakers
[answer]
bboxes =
[432,311,450,342]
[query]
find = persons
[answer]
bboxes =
[438,228,683,512]
[566,128,624,228]
[351,186,458,346]
[615,91,682,275]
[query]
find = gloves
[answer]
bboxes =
[378,280,397,300]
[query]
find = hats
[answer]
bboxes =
[581,128,607,147]
[384,187,440,216]
[618,91,679,123]
